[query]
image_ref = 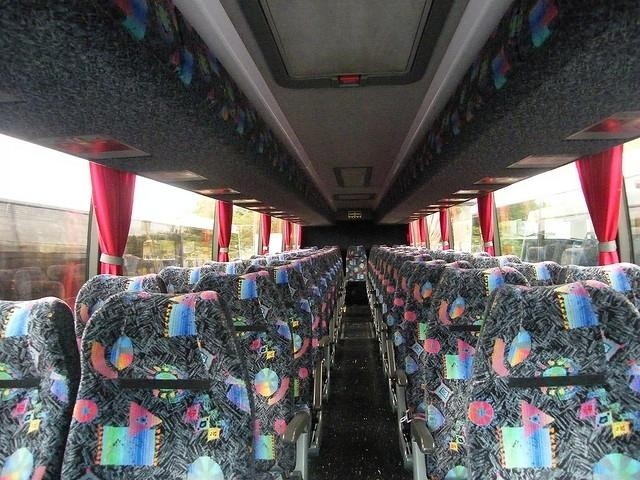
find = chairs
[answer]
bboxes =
[380,254,433,410]
[409,267,532,478]
[247,245,346,344]
[192,271,312,480]
[345,245,369,304]
[268,260,332,403]
[157,265,217,295]
[367,245,475,346]
[0,297,82,480]
[475,254,523,269]
[505,261,563,286]
[394,259,476,471]
[241,264,325,456]
[555,264,639,311]
[73,275,167,355]
[63,289,254,480]
[202,260,245,275]
[465,281,639,480]
